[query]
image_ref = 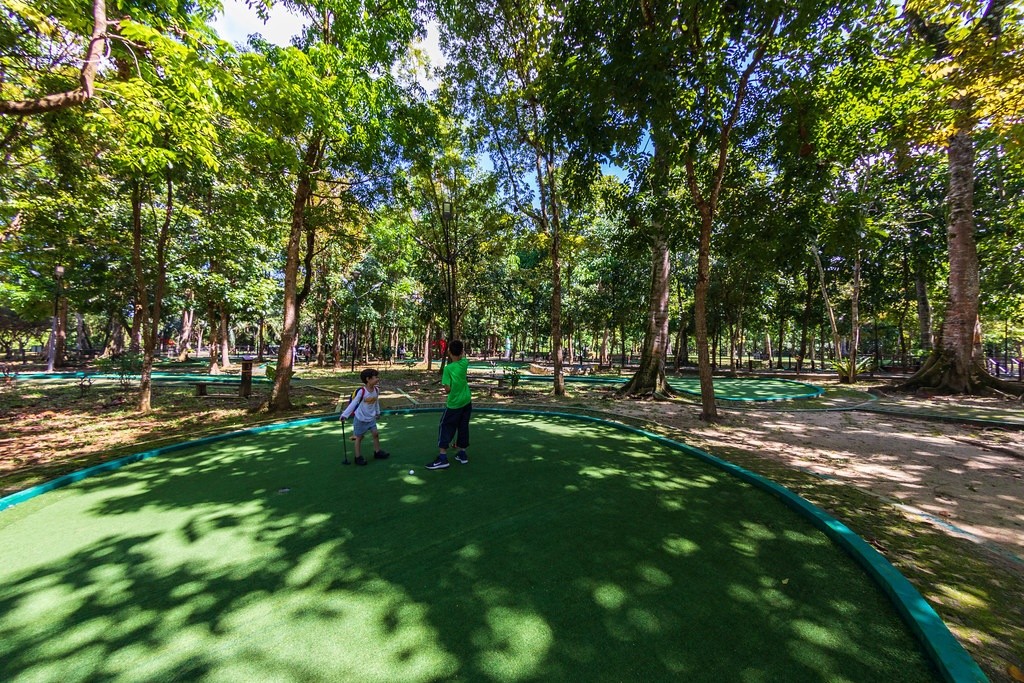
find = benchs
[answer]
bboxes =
[466,374,507,388]
[188,380,242,397]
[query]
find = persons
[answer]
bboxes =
[398,345,406,360]
[303,345,311,365]
[339,369,390,465]
[425,339,471,470]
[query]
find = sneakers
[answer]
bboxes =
[355,455,367,465]
[454,452,468,463]
[425,456,450,469]
[374,449,390,458]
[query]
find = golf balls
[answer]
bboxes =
[409,469,415,475]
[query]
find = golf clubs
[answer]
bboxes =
[339,417,352,466]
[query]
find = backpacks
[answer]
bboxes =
[350,386,378,411]
